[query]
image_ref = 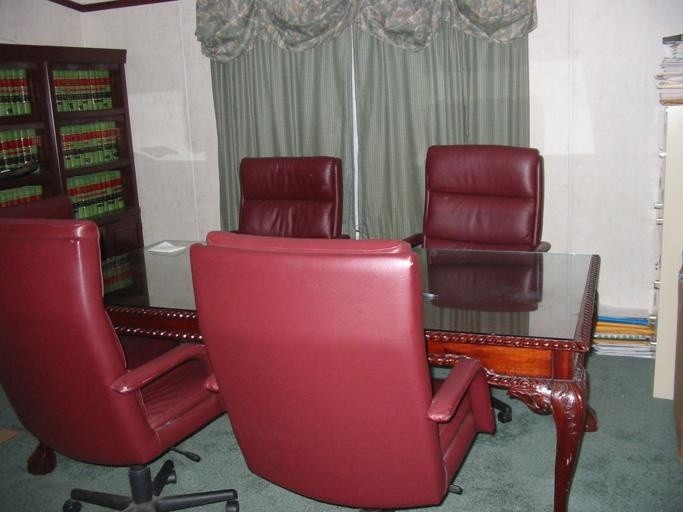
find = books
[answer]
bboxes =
[0,67,43,208]
[590,304,653,359]
[49,66,135,297]
[653,32,682,106]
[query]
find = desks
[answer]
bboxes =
[102,239,602,512]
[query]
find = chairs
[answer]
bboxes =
[402,145,551,252]
[0,197,76,475]
[0,217,240,512]
[425,246,543,310]
[186,230,498,512]
[236,157,350,240]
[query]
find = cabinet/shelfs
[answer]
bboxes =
[0,43,150,308]
[647,105,682,401]
